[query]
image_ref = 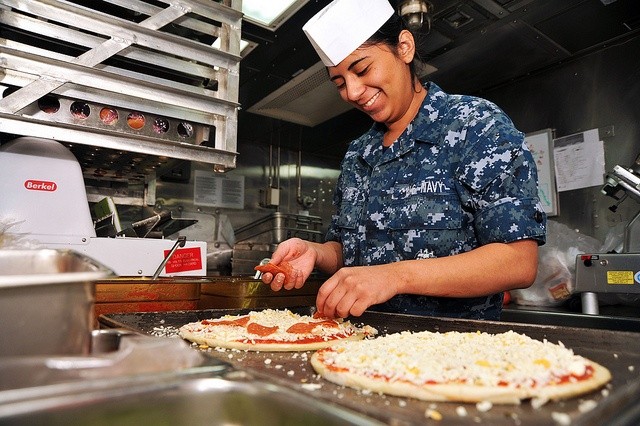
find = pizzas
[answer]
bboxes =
[311,328,612,404]
[180,308,369,353]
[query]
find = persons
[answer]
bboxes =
[261,2,546,320]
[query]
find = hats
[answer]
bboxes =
[301,1,396,68]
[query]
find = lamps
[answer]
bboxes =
[395,0,435,39]
